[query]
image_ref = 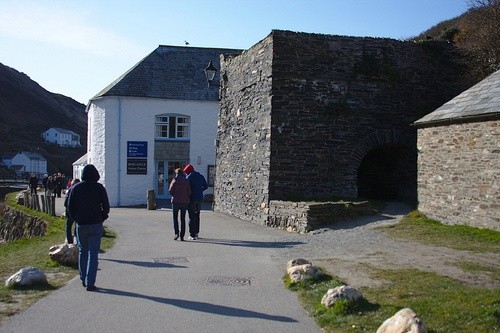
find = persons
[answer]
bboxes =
[182,165,208,240]
[168,168,192,241]
[29,171,81,244]
[67,164,109,291]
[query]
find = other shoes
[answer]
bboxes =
[174,234,179,240]
[180,235,184,241]
[87,285,97,291]
[188,236,197,240]
[196,234,198,238]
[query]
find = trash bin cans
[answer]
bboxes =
[146,189,156,210]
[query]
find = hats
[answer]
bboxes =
[183,164,194,175]
